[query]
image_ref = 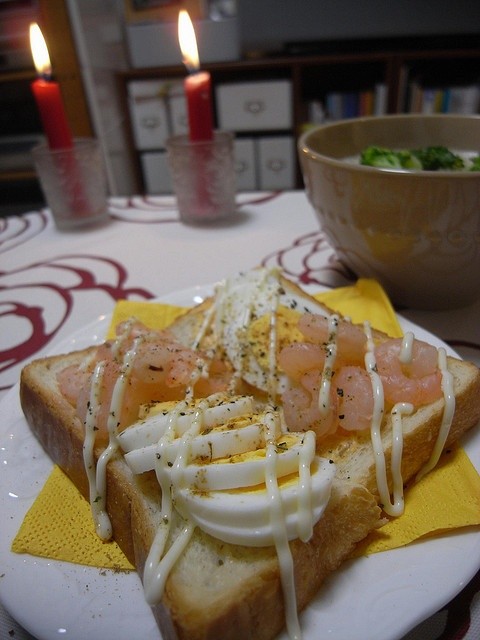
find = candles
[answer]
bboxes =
[176,11,216,215]
[29,23,89,218]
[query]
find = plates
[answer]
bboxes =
[388,307,388,308]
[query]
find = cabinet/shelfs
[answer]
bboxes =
[0,1,98,183]
[116,34,480,193]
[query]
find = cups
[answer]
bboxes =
[164,131,238,228]
[30,136,112,232]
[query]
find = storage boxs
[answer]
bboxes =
[126,14,242,67]
[258,137,295,192]
[218,83,293,129]
[234,139,257,191]
[142,155,175,193]
[130,79,189,150]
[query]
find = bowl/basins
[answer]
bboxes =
[296,112,478,307]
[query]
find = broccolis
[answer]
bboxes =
[413,143,464,170]
[357,144,411,173]
